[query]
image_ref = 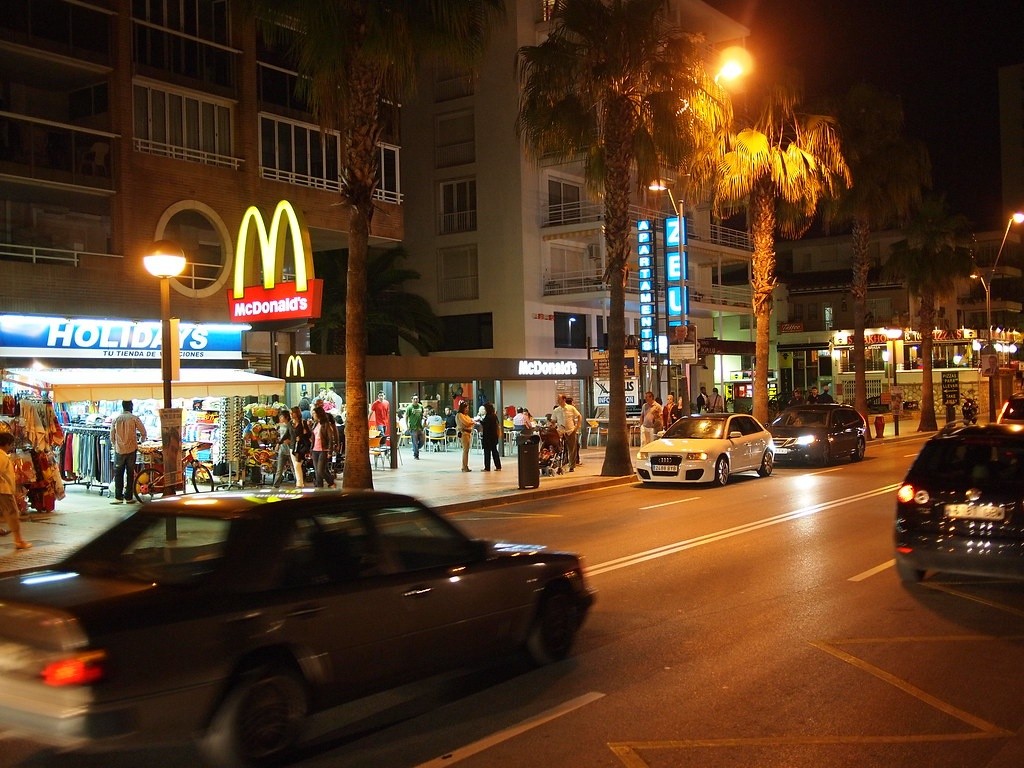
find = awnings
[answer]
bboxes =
[4,367,285,404]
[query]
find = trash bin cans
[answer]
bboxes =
[514,435,543,488]
[873,415,884,439]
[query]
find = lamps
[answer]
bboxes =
[783,352,790,360]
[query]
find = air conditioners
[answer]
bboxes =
[589,244,602,259]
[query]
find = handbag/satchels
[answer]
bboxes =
[458,431,462,438]
[707,408,713,413]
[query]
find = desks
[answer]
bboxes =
[533,417,553,425]
[575,418,640,444]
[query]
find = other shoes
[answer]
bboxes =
[481,469,489,472]
[127,499,136,503]
[569,468,573,471]
[462,467,471,472]
[15,542,32,550]
[415,457,420,460]
[0,528,11,535]
[110,499,123,504]
[494,468,501,471]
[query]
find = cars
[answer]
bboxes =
[0,486,597,768]
[635,413,775,487]
[765,403,867,467]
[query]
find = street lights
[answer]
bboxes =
[143,237,187,542]
[677,56,746,416]
[648,179,691,418]
[968,213,1024,421]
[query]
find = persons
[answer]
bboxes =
[368,390,390,436]
[474,405,487,421]
[110,401,147,505]
[640,386,723,447]
[474,402,502,472]
[397,405,462,450]
[298,386,347,462]
[267,400,339,488]
[787,388,805,405]
[537,393,582,472]
[405,392,425,460]
[984,356,998,374]
[806,387,820,405]
[453,387,473,411]
[790,412,805,425]
[476,388,489,414]
[820,386,834,403]
[454,402,480,472]
[503,406,537,444]
[497,420,501,457]
[0,431,32,549]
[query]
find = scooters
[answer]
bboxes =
[959,392,979,423]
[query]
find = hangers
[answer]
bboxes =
[61,421,112,438]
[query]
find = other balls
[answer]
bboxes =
[134,473,151,494]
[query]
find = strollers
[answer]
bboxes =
[538,424,566,477]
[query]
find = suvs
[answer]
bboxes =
[892,418,1024,585]
[996,391,1023,425]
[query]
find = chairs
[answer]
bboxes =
[369,420,643,470]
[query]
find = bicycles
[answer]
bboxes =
[133,442,214,504]
